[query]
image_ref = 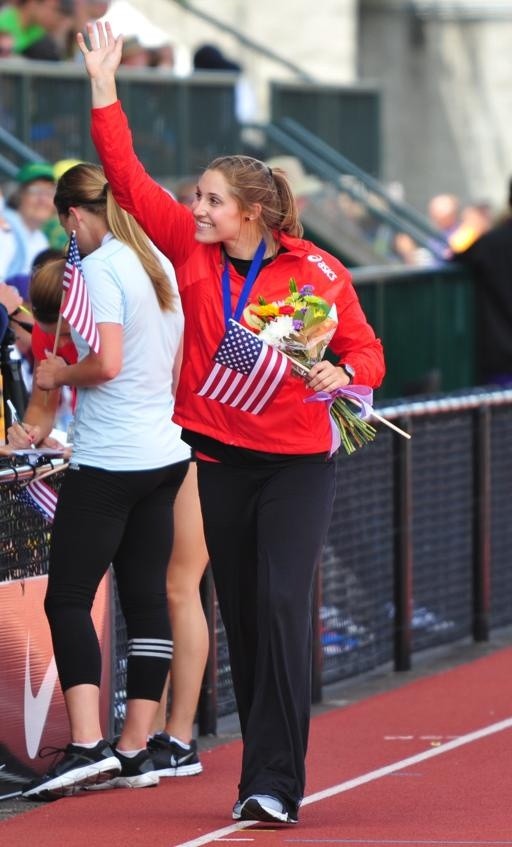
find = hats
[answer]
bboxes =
[16,156,84,187]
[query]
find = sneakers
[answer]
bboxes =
[80,747,160,791]
[147,730,203,778]
[232,797,242,820]
[21,740,123,802]
[239,793,299,824]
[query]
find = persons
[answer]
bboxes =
[1,158,210,801]
[263,154,492,263]
[0,0,175,159]
[76,19,387,825]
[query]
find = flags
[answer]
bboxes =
[190,314,294,420]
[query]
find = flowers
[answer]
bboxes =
[243,279,377,455]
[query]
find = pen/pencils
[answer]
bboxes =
[6,399,35,449]
